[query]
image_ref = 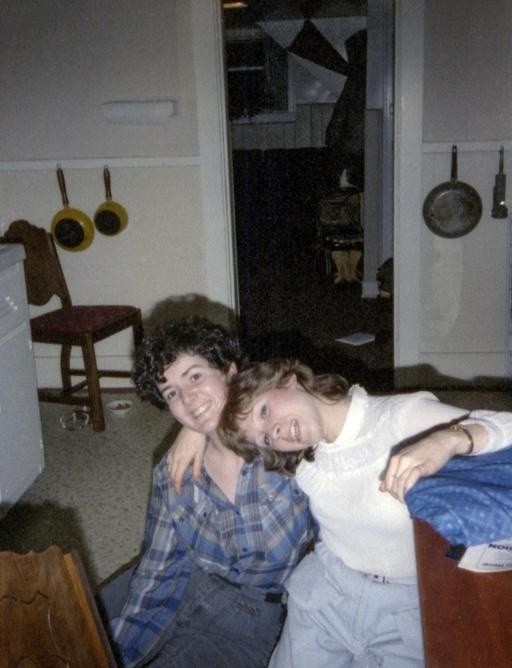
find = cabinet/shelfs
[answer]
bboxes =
[0,244,47,527]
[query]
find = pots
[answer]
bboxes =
[44,163,127,253]
[422,145,483,239]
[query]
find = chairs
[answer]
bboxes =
[0,219,142,433]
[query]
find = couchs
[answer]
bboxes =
[97,449,512,667]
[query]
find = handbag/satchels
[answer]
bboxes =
[403,445,512,548]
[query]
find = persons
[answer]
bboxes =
[97,315,318,668]
[168,359,512,668]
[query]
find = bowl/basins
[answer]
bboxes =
[107,400,133,415]
[60,405,91,431]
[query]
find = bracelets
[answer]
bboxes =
[449,425,473,454]
